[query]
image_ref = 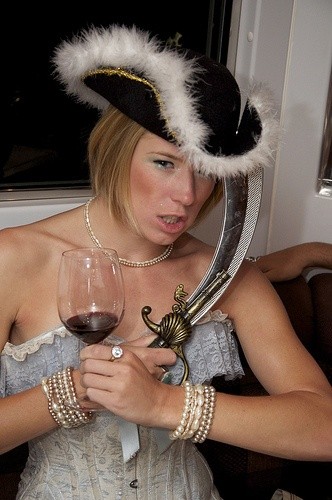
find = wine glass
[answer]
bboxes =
[57,247,125,411]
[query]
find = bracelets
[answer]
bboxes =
[40,365,96,430]
[168,380,216,445]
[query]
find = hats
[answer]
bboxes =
[52,24,282,179]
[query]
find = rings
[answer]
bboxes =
[247,256,261,263]
[109,345,124,361]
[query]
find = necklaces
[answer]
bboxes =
[83,192,175,268]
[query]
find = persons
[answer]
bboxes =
[0,23,332,500]
[246,242,332,282]
